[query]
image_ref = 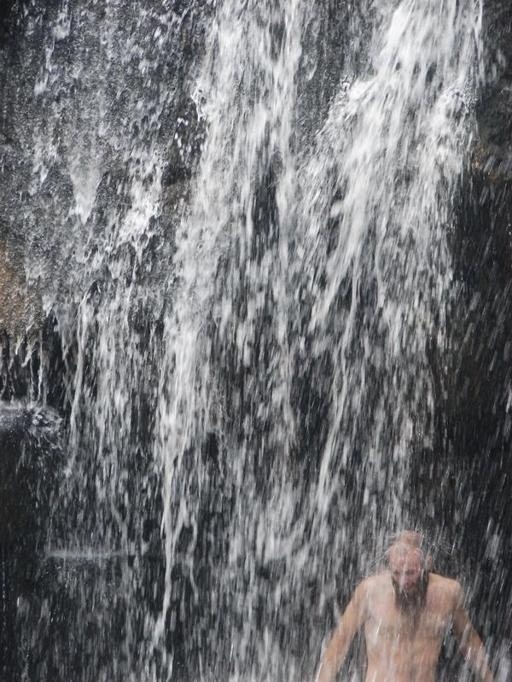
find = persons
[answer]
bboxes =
[315,531,495,682]
